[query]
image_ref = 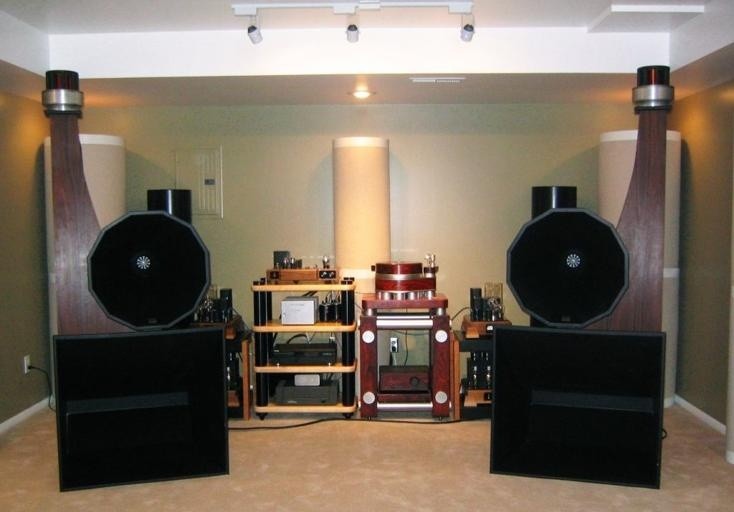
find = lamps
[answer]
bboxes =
[230,1,476,43]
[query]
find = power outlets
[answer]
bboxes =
[23,355,31,375]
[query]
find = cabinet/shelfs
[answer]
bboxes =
[357,293,452,421]
[228,328,253,423]
[455,330,495,422]
[252,275,360,419]
